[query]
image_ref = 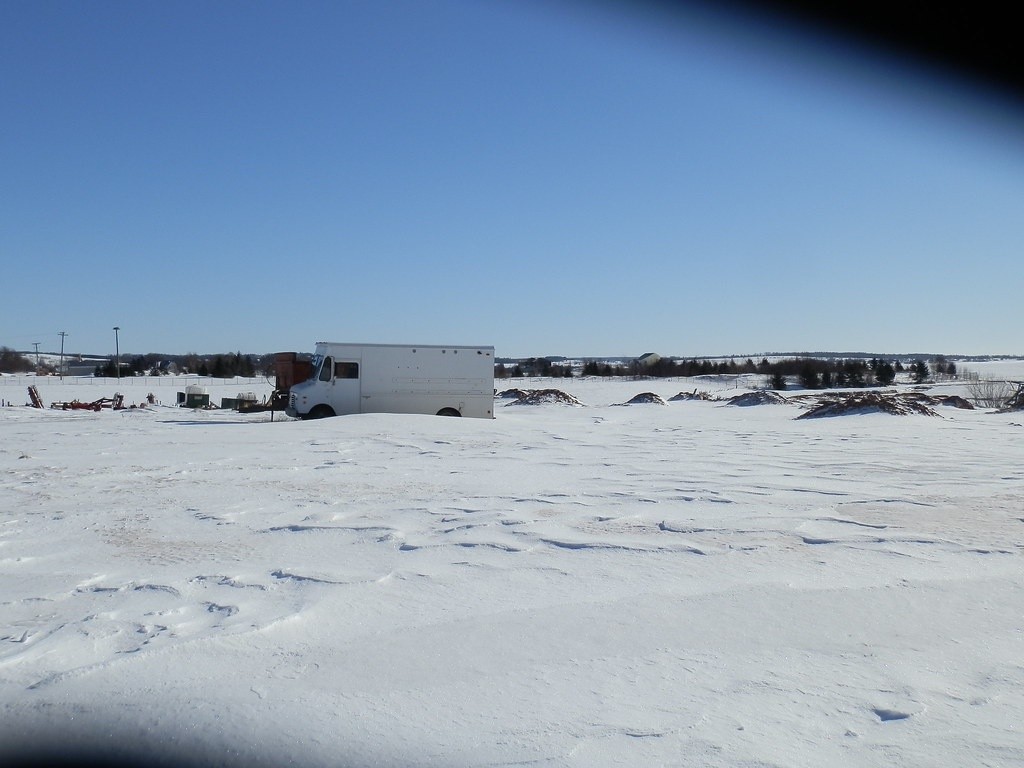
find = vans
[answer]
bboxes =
[284,341,496,422]
